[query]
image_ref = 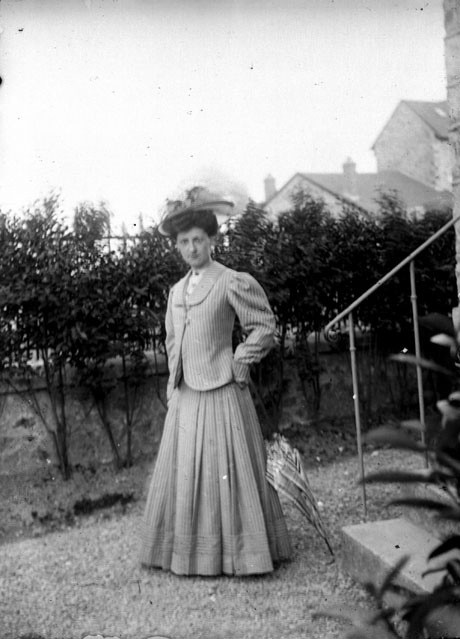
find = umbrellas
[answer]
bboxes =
[244,374,336,557]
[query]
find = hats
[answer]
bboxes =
[158,187,235,237]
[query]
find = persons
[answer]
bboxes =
[137,207,293,576]
